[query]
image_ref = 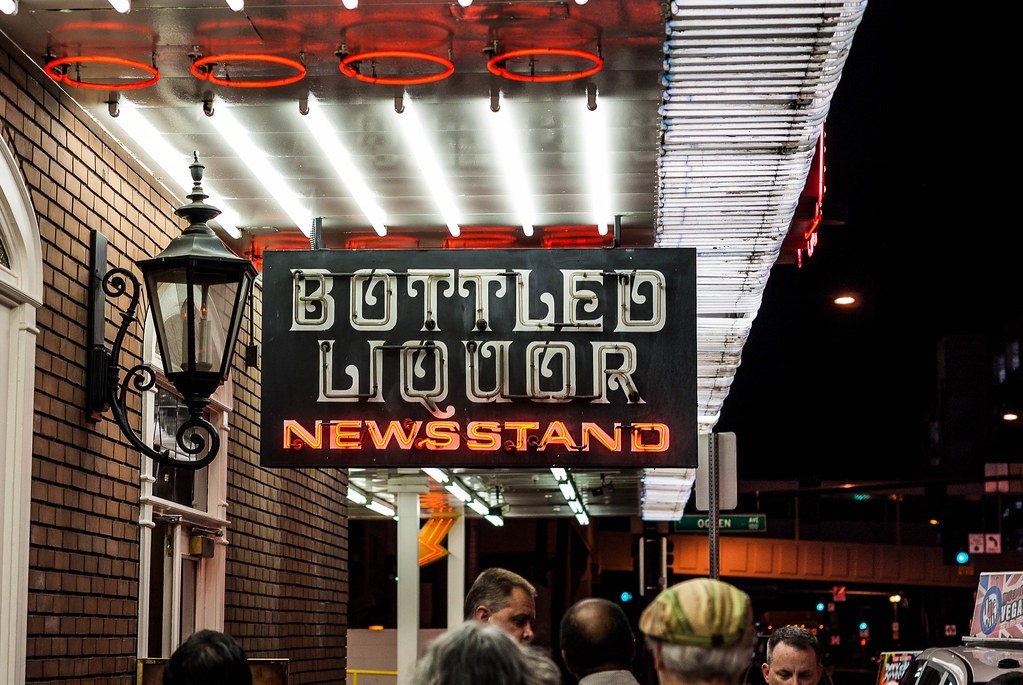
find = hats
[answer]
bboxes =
[638,577,753,647]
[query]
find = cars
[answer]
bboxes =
[896,645,1023,685]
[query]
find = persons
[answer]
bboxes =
[162,629,253,685]
[404,568,823,685]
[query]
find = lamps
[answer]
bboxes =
[483,486,505,526]
[86,150,259,470]
